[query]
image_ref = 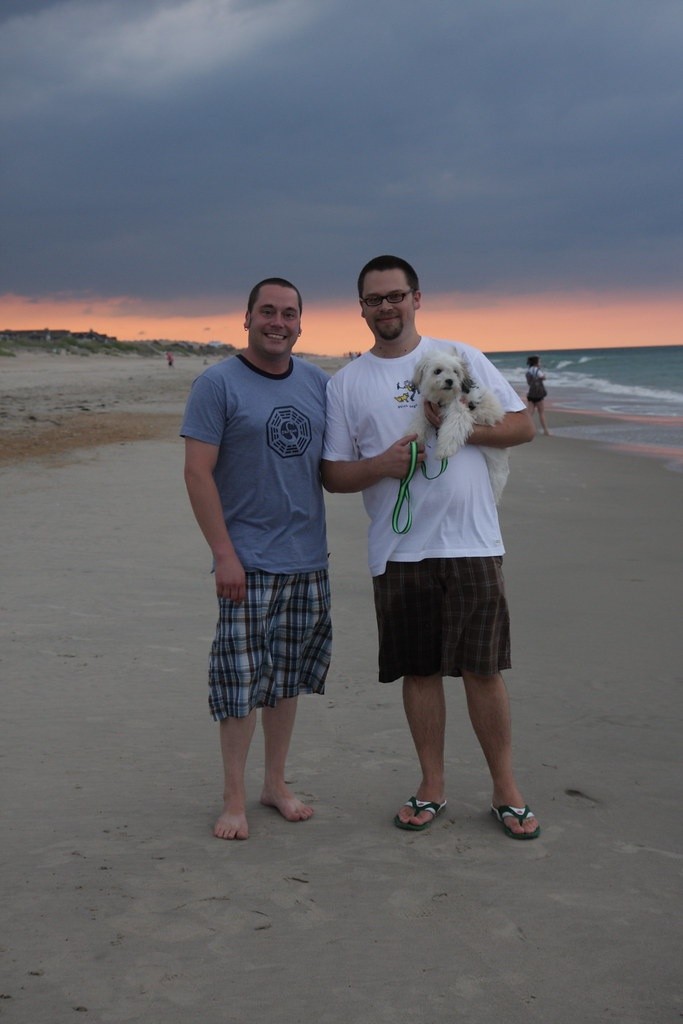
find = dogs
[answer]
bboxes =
[413,351,509,505]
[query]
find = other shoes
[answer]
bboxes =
[544,432,553,436]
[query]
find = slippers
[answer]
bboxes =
[395,796,447,830]
[491,803,541,838]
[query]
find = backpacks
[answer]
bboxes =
[526,371,534,385]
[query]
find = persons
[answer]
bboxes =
[320,255,546,844]
[524,355,553,436]
[181,278,332,839]
[165,351,176,369]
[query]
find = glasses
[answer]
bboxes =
[360,289,414,306]
